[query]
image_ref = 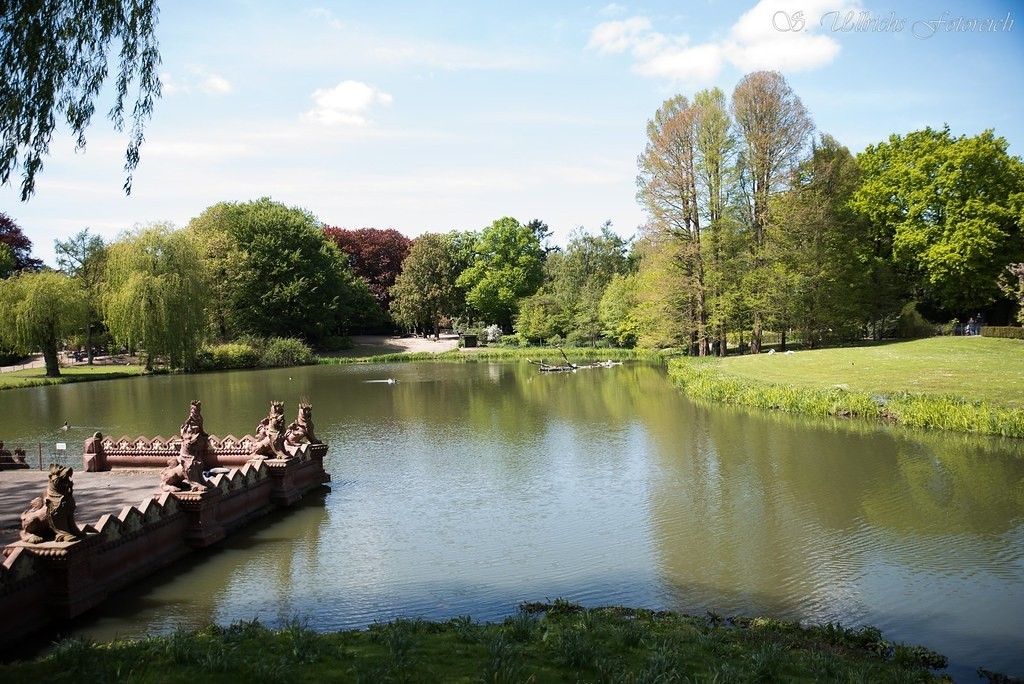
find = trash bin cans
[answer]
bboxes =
[462,334,479,347]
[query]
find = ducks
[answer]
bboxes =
[62,420,71,428]
[388,377,397,384]
[288,377,292,380]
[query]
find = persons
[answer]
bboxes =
[966,313,982,335]
[0,440,30,469]
